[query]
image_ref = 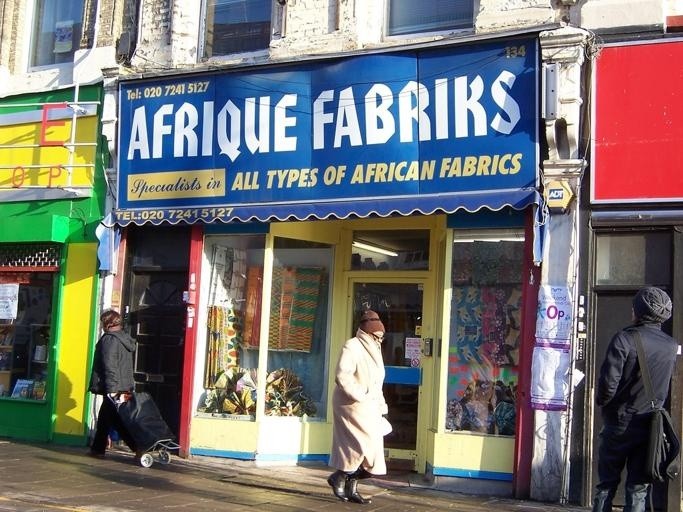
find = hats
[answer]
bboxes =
[359,310,386,334]
[632,286,672,324]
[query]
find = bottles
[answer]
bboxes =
[33,333,47,361]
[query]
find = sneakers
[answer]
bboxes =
[86,448,104,459]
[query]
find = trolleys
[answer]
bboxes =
[107,392,183,469]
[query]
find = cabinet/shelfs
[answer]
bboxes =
[0,322,50,395]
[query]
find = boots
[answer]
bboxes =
[327,470,349,501]
[346,478,372,504]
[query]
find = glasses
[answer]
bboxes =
[374,334,385,342]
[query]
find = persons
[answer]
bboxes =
[326,310,393,504]
[84,308,147,462]
[588,284,677,511]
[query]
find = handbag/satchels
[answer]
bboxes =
[648,407,681,481]
